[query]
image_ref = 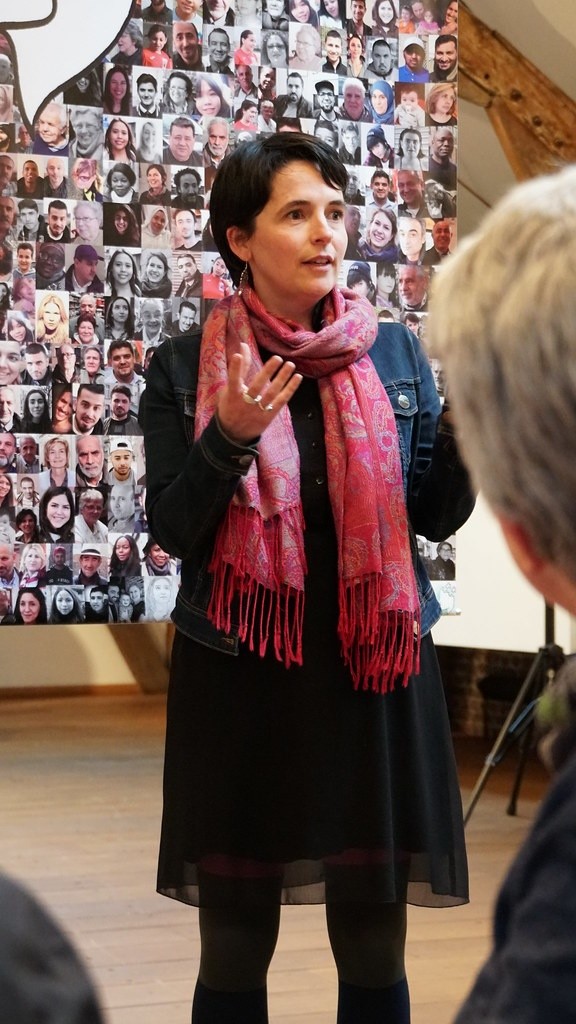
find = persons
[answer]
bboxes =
[276,70,311,119]
[66,244,104,293]
[136,131,476,1024]
[103,65,133,116]
[104,162,138,203]
[105,250,143,297]
[170,168,204,208]
[347,35,367,80]
[233,28,261,65]
[161,73,202,115]
[310,77,342,120]
[291,23,320,71]
[173,254,203,298]
[0,0,456,627]
[173,21,204,71]
[426,162,576,1023]
[42,200,69,243]
[164,117,203,164]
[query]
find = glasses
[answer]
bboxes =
[318,92,332,96]
[73,217,97,223]
[38,252,64,264]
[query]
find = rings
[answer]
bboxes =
[260,404,273,413]
[243,391,263,404]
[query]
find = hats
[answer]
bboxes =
[315,79,334,91]
[75,245,105,262]
[367,126,385,140]
[109,438,135,455]
[349,262,371,279]
[54,547,66,556]
[73,543,105,560]
[402,36,425,52]
